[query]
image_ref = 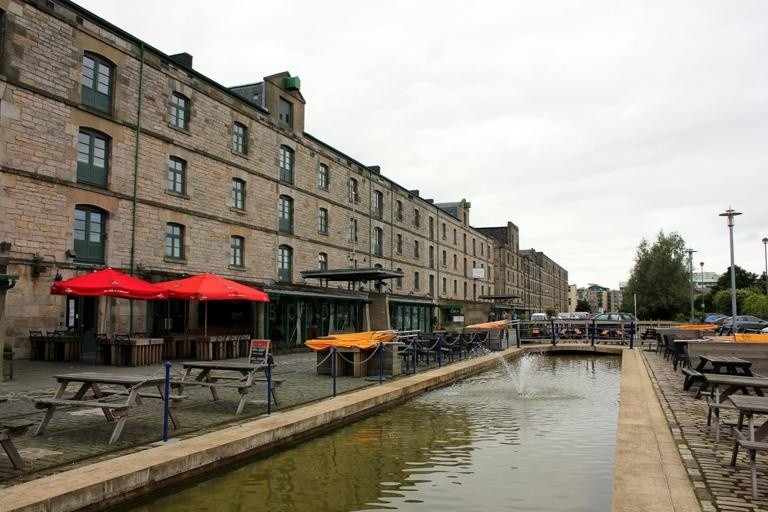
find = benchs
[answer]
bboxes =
[639,325,768,503]
[1,399,35,471]
[31,363,285,445]
[548,326,630,348]
[399,329,487,373]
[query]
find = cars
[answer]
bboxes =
[531,309,640,335]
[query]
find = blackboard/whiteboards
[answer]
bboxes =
[249,339,271,361]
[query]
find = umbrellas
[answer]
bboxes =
[50,267,168,334]
[153,272,270,336]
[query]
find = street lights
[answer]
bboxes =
[683,203,767,334]
